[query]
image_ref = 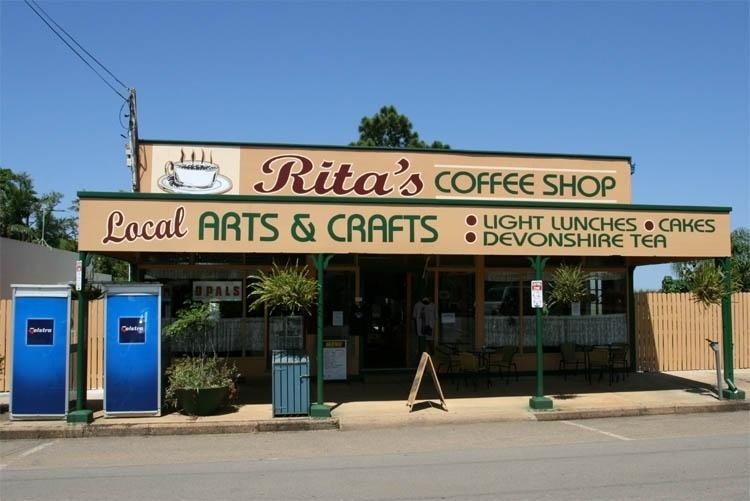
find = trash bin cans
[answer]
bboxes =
[272,349,310,416]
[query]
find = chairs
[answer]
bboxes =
[555,341,631,387]
[437,343,520,392]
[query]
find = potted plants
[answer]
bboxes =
[542,263,606,316]
[162,352,242,420]
